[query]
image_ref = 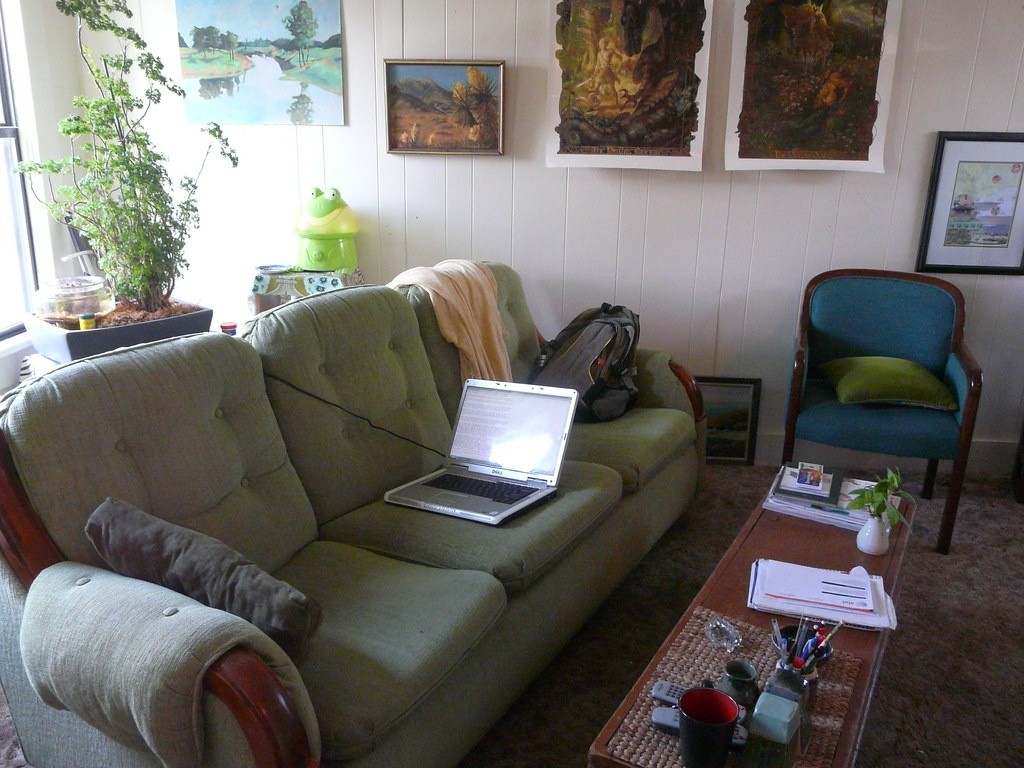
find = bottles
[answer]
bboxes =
[715,659,759,710]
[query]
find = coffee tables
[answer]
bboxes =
[582,465,919,768]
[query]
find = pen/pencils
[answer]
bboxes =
[771,618,782,646]
[802,637,817,660]
[811,504,850,516]
[802,645,823,675]
[805,621,824,644]
[796,619,810,656]
[781,638,788,667]
[818,620,844,647]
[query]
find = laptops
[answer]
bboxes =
[384,378,580,527]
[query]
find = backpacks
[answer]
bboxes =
[528,302,640,421]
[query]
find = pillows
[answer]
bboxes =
[819,354,956,408]
[81,497,321,658]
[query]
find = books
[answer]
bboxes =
[761,461,898,534]
[746,560,894,631]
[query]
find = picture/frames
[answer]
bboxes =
[380,58,507,158]
[915,131,1024,277]
[696,373,763,466]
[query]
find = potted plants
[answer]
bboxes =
[842,468,911,553]
[23,0,238,362]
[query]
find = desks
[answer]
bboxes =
[252,265,362,316]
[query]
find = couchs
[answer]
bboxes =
[1,259,701,768]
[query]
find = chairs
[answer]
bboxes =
[788,266,986,558]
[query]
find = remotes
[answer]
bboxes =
[650,680,748,747]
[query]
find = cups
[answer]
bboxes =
[679,679,739,768]
